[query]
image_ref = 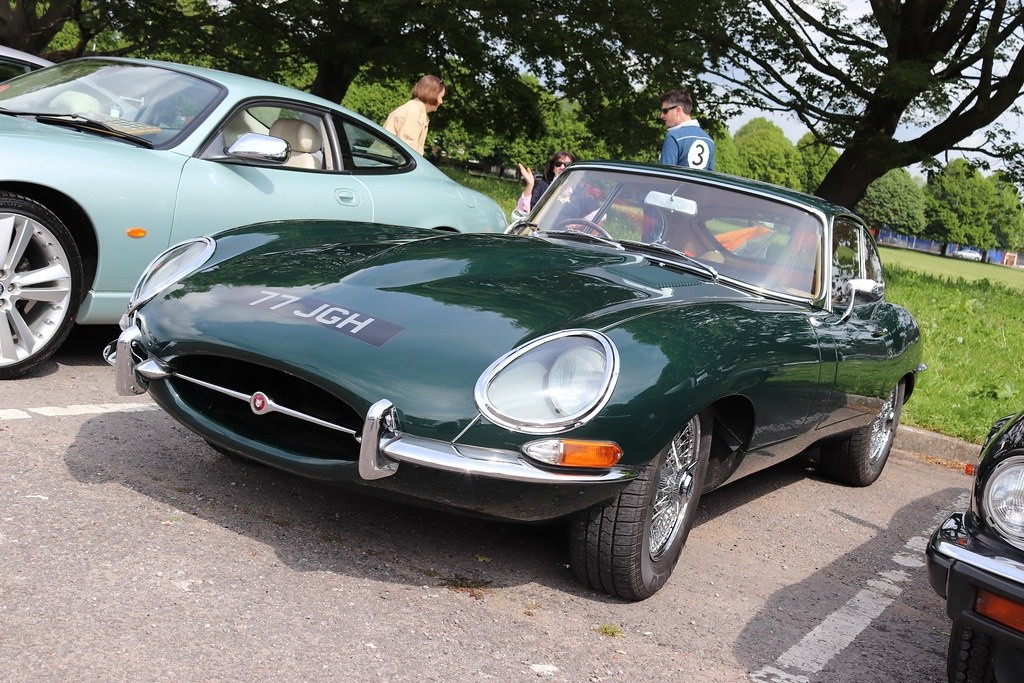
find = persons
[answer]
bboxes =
[368,74,445,161]
[517,151,607,231]
[641,91,714,253]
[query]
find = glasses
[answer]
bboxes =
[440,79,444,84]
[553,160,572,168]
[662,104,681,114]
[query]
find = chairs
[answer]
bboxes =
[47,91,100,114]
[268,118,323,170]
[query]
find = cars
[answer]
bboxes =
[0,56,511,380]
[0,46,166,128]
[103,161,927,605]
[953,250,983,262]
[882,236,906,245]
[926,410,1024,683]
